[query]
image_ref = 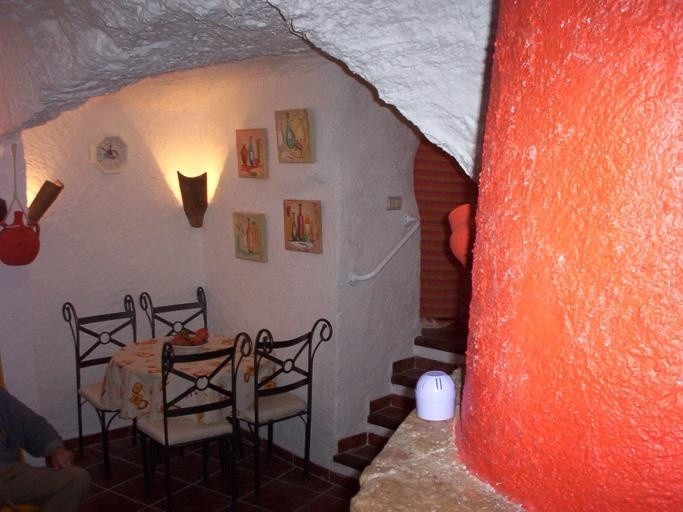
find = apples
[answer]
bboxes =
[173,328,209,346]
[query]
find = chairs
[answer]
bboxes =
[227,318,333,495]
[139,286,207,459]
[0,352,41,512]
[61,294,157,480]
[141,329,253,511]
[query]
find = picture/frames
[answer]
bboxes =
[235,127,269,180]
[232,211,268,263]
[273,107,316,163]
[283,199,323,254]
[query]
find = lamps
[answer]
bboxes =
[177,170,209,228]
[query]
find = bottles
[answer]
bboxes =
[291,203,312,244]
[238,217,257,253]
[240,135,261,167]
[277,113,296,150]
[0,209,40,265]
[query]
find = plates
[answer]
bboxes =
[161,335,209,348]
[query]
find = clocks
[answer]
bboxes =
[89,133,130,173]
